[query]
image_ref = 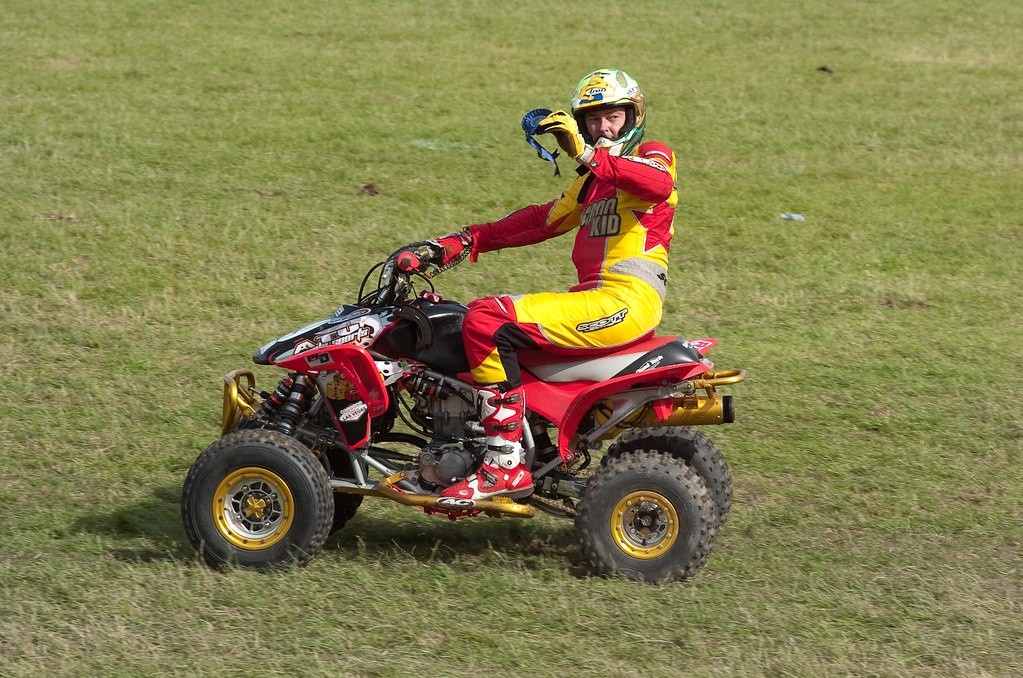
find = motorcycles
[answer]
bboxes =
[181,243,747,583]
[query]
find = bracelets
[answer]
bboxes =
[576,143,594,165]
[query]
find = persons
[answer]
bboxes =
[418,70,679,514]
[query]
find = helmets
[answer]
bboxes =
[571,68,647,160]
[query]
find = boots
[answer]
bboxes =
[441,383,534,503]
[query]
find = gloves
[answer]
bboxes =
[536,109,585,159]
[428,228,473,277]
